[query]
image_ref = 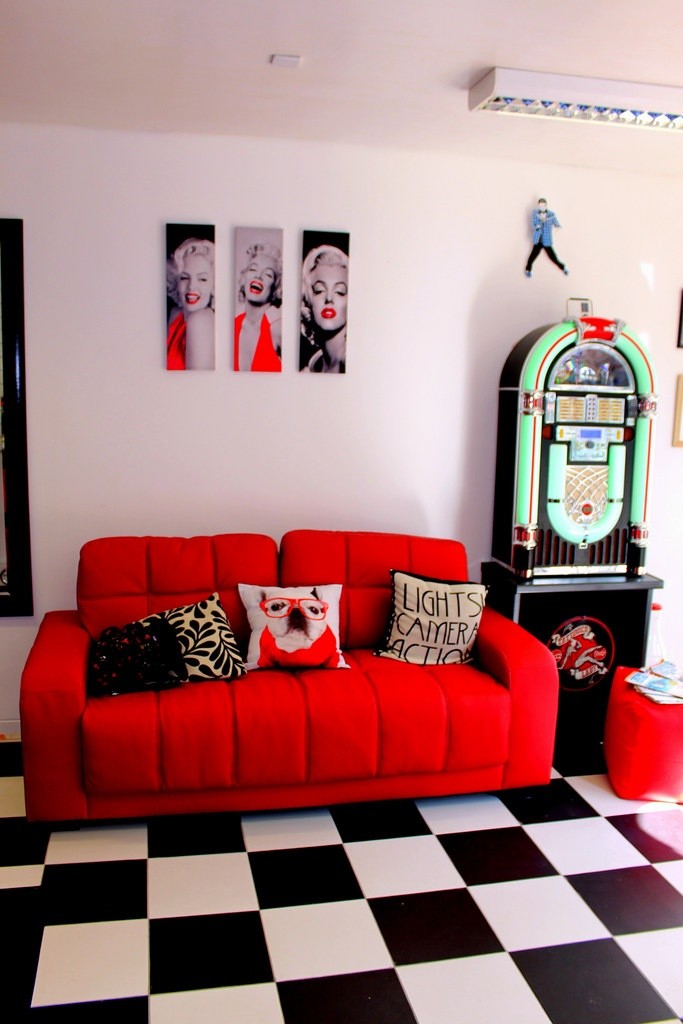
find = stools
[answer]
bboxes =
[601,664,683,802]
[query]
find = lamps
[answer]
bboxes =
[467,68,683,137]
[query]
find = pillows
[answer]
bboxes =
[137,592,249,683]
[372,568,493,664]
[236,582,353,670]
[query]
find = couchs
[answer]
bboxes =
[18,530,558,833]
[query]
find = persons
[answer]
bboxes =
[166,238,215,371]
[300,245,349,374]
[525,198,569,277]
[234,242,282,373]
